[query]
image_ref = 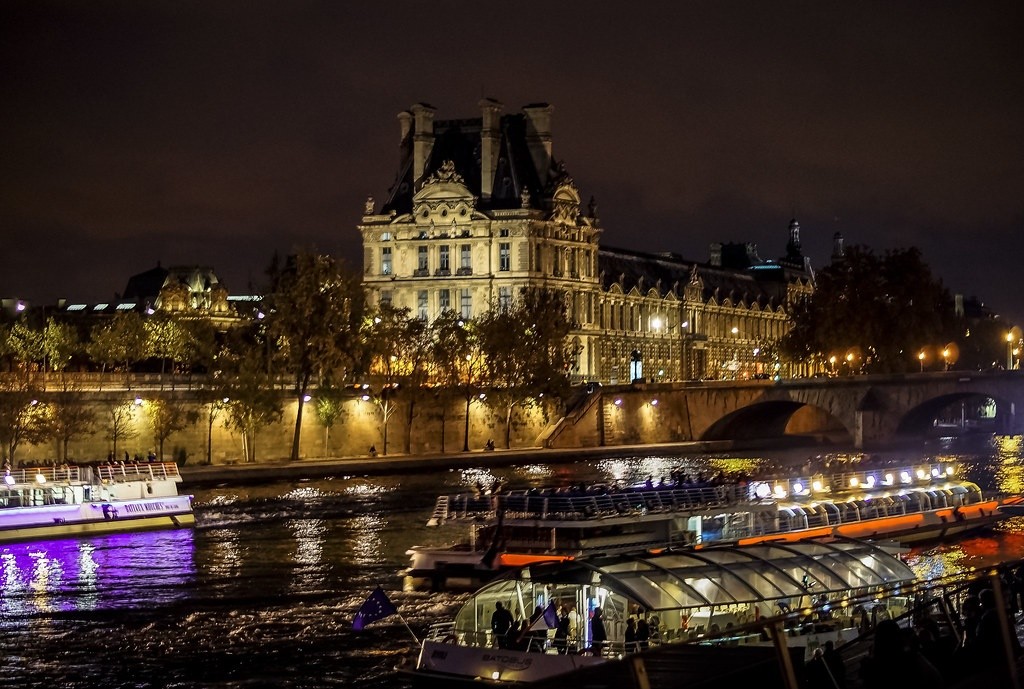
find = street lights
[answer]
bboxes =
[919,352,924,371]
[653,319,688,381]
[942,350,949,371]
[1004,332,1015,370]
[846,354,856,374]
[731,328,761,379]
[829,355,836,376]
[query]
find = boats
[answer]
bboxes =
[1,457,197,541]
[350,459,1023,689]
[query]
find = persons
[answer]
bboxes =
[454,438,937,513]
[492,595,830,658]
[369,444,376,457]
[5,450,158,480]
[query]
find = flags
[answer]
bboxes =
[544,602,559,630]
[352,586,399,630]
